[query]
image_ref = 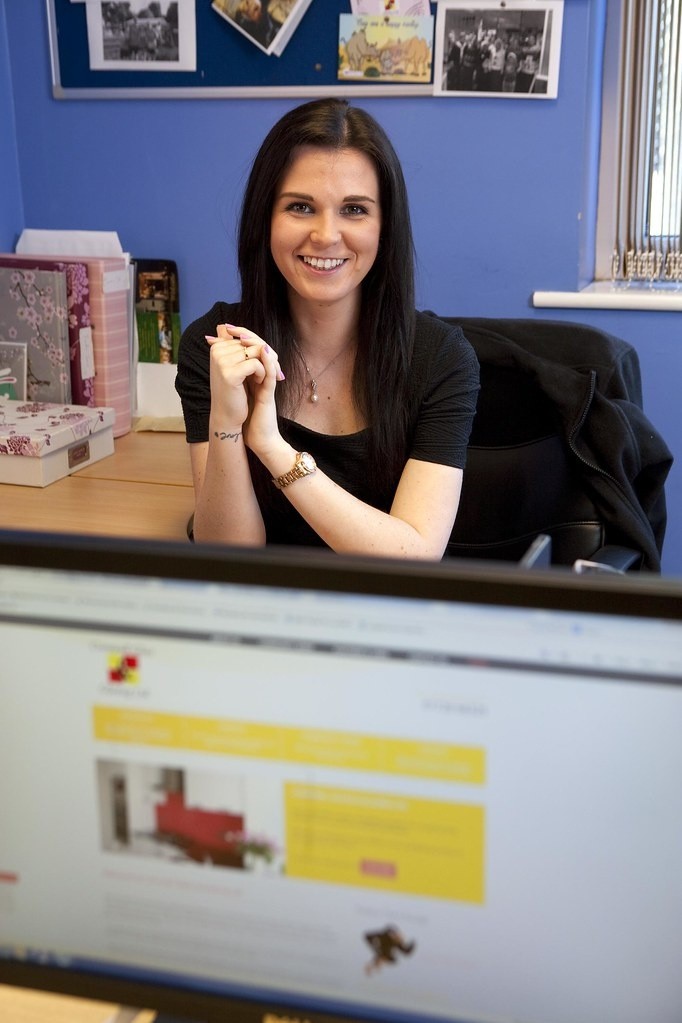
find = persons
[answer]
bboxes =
[446,20,543,93]
[174,98,479,564]
[118,16,163,62]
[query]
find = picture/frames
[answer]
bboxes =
[433,0,564,100]
[85,0,196,72]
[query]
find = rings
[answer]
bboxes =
[243,346,250,360]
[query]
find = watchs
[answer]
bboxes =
[272,451,316,490]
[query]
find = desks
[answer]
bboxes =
[0,414,197,544]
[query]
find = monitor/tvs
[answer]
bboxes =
[0,527,681,1023]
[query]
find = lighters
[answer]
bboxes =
[288,328,358,402]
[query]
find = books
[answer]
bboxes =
[0,253,141,439]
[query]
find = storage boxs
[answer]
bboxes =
[0,400,116,488]
